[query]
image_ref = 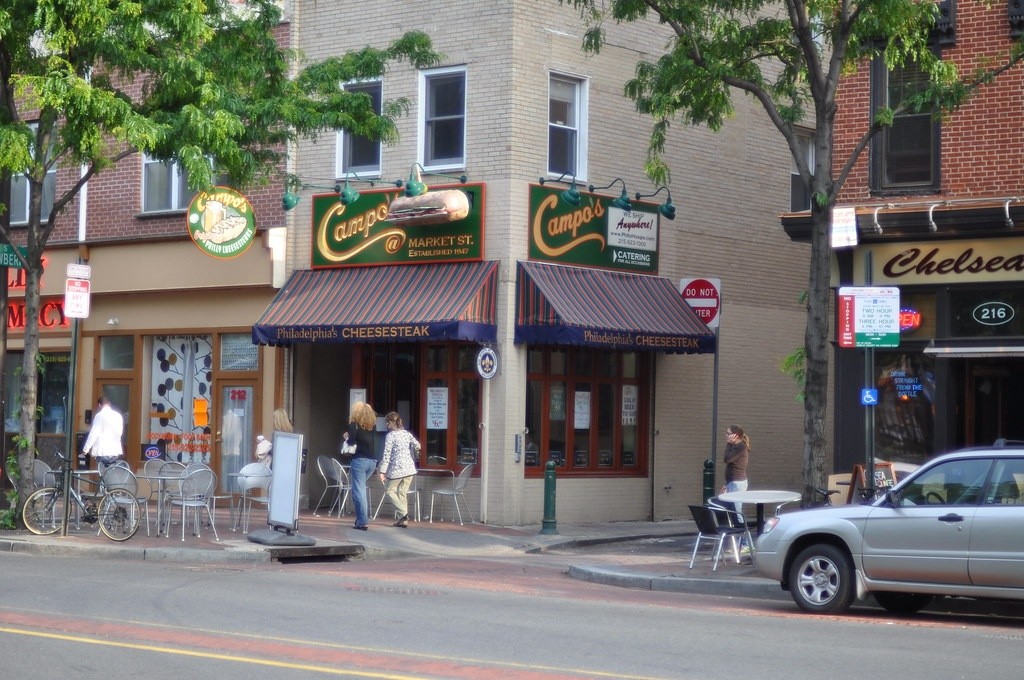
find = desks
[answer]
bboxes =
[718,490,801,538]
[415,468,450,519]
[159,469,186,472]
[136,474,188,537]
[42,470,99,530]
[228,473,271,534]
[341,465,351,474]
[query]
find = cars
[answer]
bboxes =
[749,437,1024,618]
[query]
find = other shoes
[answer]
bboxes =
[393,521,408,528]
[397,515,410,525]
[740,545,755,553]
[726,548,734,553]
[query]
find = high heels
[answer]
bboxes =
[353,524,368,531]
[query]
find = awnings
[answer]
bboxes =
[514,260,716,355]
[252,259,500,348]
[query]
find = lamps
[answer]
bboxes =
[539,172,582,208]
[340,170,403,207]
[928,204,938,232]
[636,187,675,220]
[282,180,342,211]
[404,163,467,197]
[107,318,119,325]
[589,179,631,212]
[1004,199,1015,230]
[873,207,884,236]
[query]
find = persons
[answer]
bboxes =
[256,408,292,455]
[721,425,755,554]
[342,402,378,531]
[378,412,421,528]
[82,397,123,468]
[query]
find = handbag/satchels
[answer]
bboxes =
[410,442,421,462]
[341,422,359,456]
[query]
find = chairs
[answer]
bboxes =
[313,455,371,519]
[372,461,421,522]
[688,496,756,572]
[5,459,273,543]
[430,464,475,525]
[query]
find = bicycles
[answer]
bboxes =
[20,449,142,542]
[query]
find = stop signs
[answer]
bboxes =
[679,277,722,329]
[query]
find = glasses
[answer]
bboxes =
[726,432,735,436]
